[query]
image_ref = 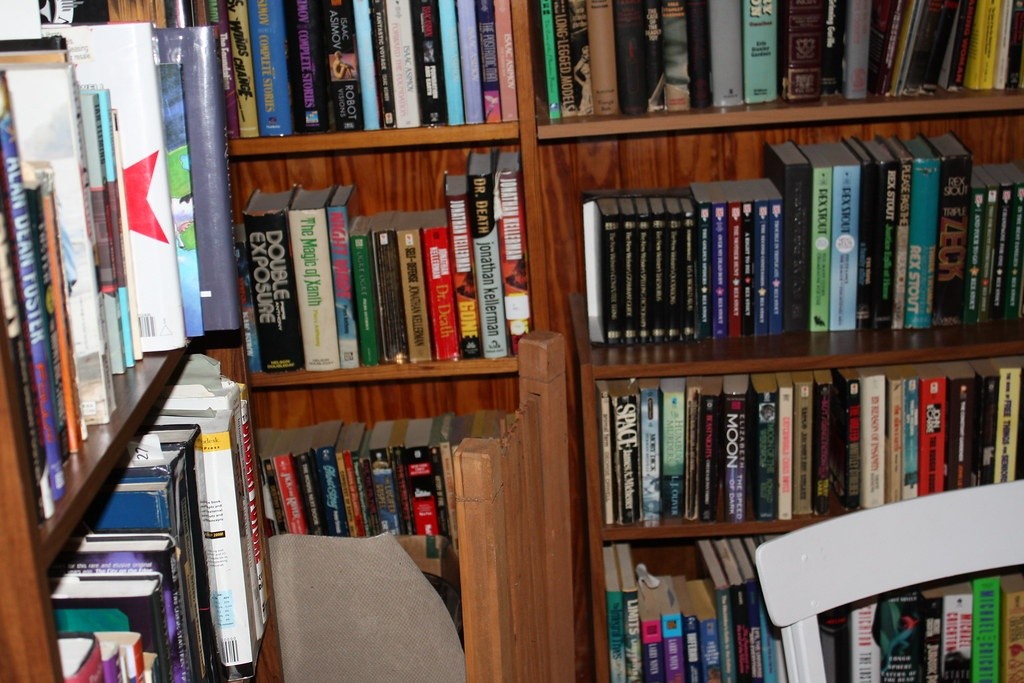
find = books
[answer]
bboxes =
[207,0,1024,139]
[235,129,1024,374]
[0,0,270,683]
[252,355,1024,683]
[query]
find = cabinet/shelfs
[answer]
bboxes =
[0,0,1024,683]
